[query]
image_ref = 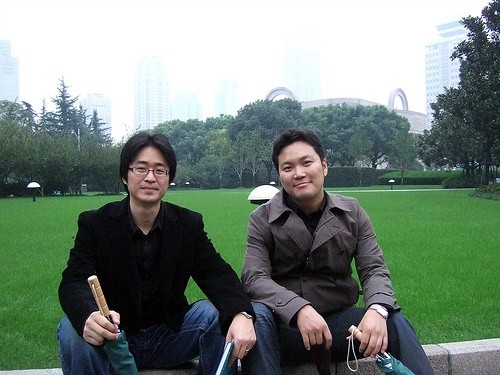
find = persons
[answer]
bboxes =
[58,132,257,375]
[240,128,435,375]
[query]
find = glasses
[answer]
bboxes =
[128,166,171,177]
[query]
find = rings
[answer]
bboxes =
[245,349,250,352]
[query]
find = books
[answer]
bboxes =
[215,342,238,375]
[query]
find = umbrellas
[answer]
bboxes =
[87,274,140,375]
[347,323,416,375]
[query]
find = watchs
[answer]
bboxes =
[240,311,254,319]
[370,304,389,320]
